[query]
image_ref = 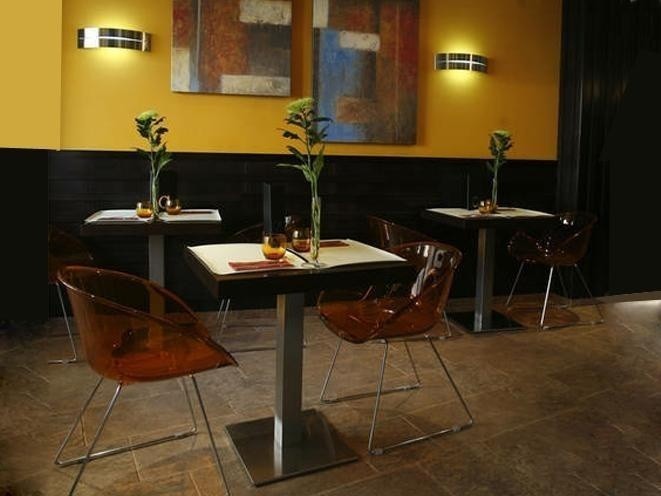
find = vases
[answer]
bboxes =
[148,170,163,221]
[301,196,327,268]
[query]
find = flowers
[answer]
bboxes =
[277,94,337,256]
[484,131,515,205]
[129,110,176,211]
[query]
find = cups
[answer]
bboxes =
[136,202,153,218]
[292,227,312,252]
[486,199,493,211]
[261,232,289,260]
[166,197,182,216]
[478,202,488,214]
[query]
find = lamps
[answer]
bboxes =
[434,53,489,79]
[76,27,153,54]
